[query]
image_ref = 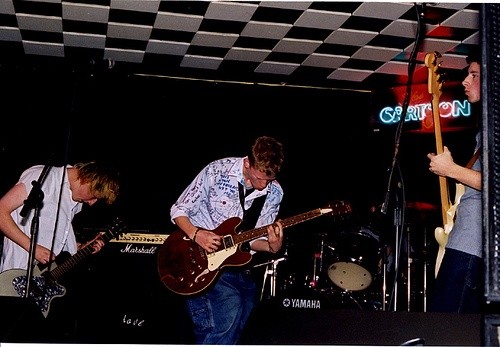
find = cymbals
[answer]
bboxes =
[375,201,436,211]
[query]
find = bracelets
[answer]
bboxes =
[193,228,200,243]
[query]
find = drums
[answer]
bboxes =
[253,275,377,311]
[327,232,381,292]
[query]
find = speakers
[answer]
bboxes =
[312,307,482,346]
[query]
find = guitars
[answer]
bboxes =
[424,50,466,280]
[0,217,129,319]
[156,199,353,295]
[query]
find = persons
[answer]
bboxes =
[426,48,483,314]
[0,161,121,279]
[170,136,284,345]
